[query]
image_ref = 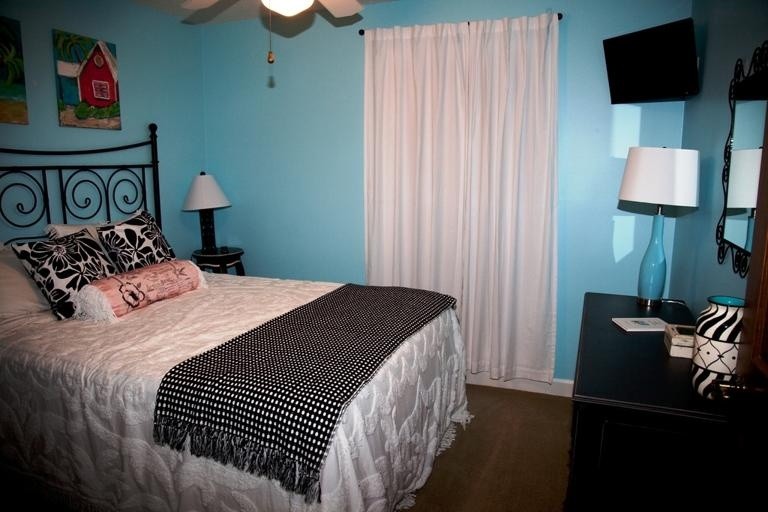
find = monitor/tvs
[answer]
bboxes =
[602,16,701,105]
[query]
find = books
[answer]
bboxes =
[664,323,696,359]
[611,317,668,332]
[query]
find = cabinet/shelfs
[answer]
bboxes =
[568,292,727,511]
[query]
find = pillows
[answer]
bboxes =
[11,228,119,321]
[42,223,117,274]
[96,211,176,273]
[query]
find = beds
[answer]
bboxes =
[1,122,474,512]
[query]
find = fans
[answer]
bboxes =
[180,0,364,18]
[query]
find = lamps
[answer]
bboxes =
[261,0,317,17]
[184,171,232,252]
[619,146,700,306]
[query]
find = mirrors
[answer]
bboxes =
[722,99,768,257]
